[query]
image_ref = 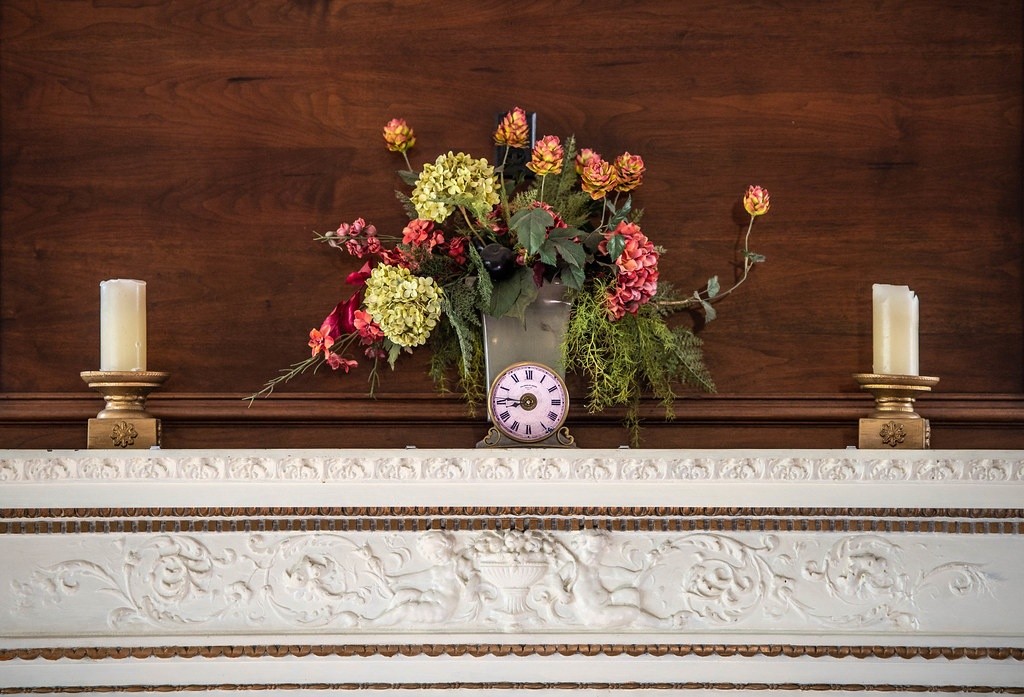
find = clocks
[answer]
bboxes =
[488,359,570,444]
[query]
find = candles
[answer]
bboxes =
[100,279,147,371]
[872,283,920,377]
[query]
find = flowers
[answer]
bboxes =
[241,106,771,448]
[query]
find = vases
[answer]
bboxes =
[476,275,576,448]
